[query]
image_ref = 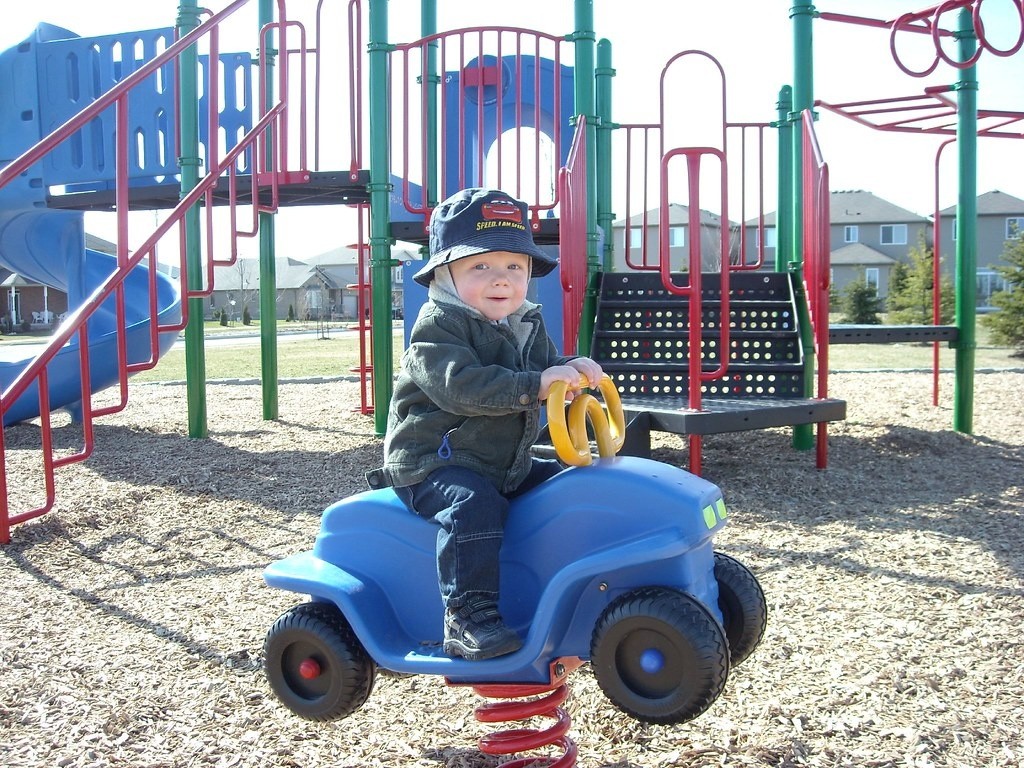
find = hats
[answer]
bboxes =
[412,188,558,289]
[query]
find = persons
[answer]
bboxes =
[383,188,601,660]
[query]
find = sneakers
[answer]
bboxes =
[442,596,522,661]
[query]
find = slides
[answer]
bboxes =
[0,217,183,426]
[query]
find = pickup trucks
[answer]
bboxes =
[12,316,52,334]
